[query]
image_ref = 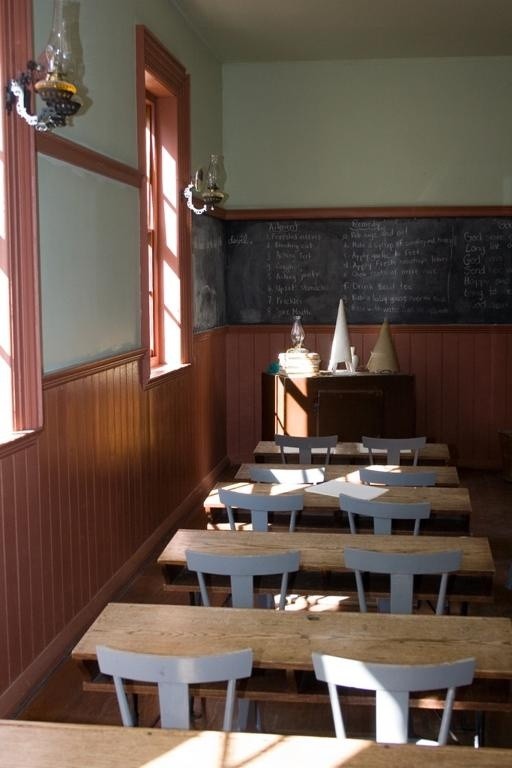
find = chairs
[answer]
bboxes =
[94,643,254,732]
[311,651,476,746]
[343,546,464,616]
[234,438,460,488]
[217,487,304,532]
[185,548,301,611]
[338,492,431,536]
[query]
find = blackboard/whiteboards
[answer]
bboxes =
[187,205,512,338]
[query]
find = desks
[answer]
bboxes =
[203,481,471,536]
[157,527,495,603]
[71,599,512,714]
[0,717,512,768]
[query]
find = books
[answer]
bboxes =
[277,351,322,378]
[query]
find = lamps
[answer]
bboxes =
[6,0,84,134]
[184,153,224,215]
